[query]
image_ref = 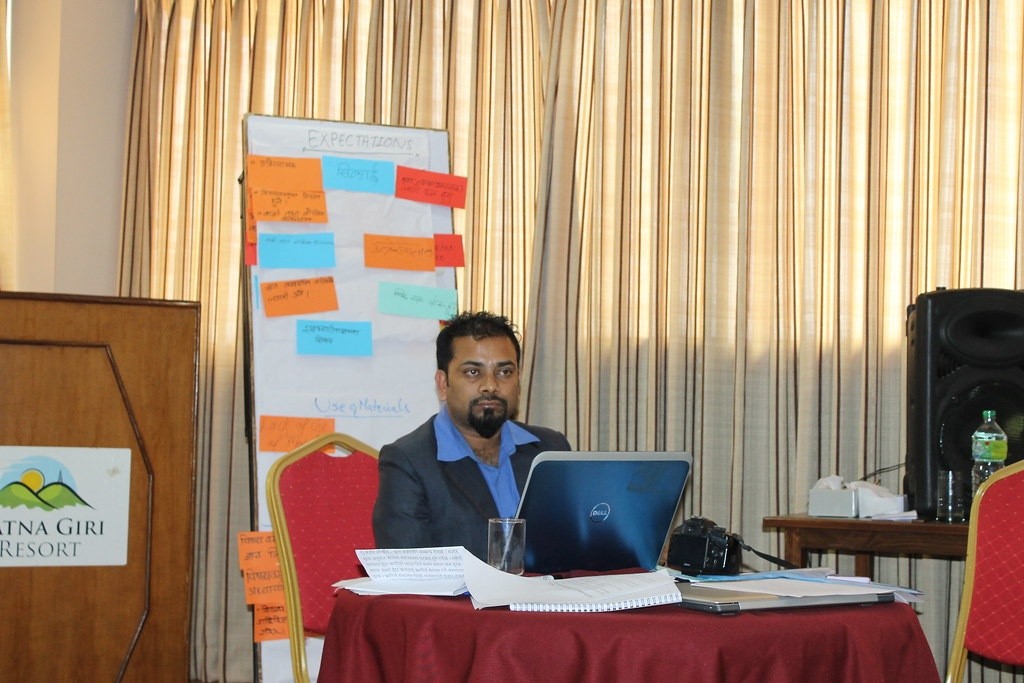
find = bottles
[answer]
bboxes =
[971,410,1008,499]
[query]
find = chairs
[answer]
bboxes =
[946,461,1024,683]
[266,432,379,683]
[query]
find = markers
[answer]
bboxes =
[529,574,562,582]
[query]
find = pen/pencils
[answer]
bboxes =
[657,564,696,583]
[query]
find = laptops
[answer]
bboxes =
[496,451,693,571]
[673,581,895,613]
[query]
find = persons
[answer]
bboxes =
[372,311,670,567]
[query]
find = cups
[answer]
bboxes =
[488,518,527,576]
[936,469,966,522]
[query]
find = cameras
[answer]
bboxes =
[667,517,742,574]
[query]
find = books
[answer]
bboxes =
[508,569,683,612]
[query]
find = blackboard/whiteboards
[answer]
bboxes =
[241,116,459,682]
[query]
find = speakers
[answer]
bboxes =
[904,287,1024,520]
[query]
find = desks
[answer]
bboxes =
[317,570,943,683]
[763,513,969,583]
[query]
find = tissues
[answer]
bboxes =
[807,474,859,519]
